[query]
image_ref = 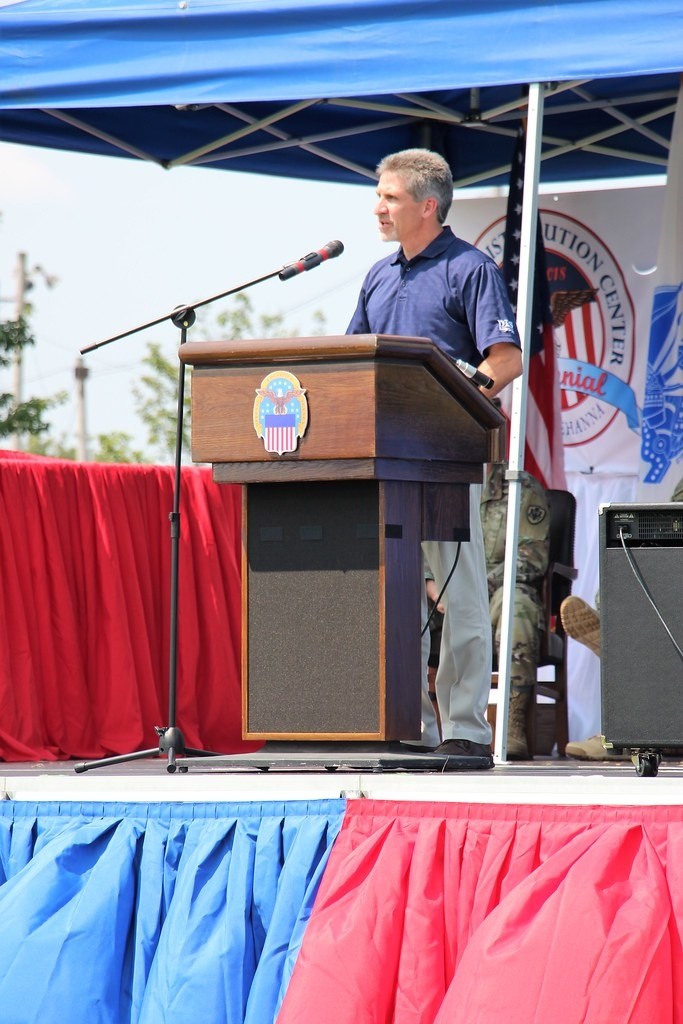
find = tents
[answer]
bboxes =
[0,0,683,185]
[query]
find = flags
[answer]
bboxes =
[634,85,683,502]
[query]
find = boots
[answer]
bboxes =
[509,694,534,762]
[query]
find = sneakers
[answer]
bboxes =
[560,595,601,656]
[565,734,631,760]
[348,742,437,771]
[426,740,496,770]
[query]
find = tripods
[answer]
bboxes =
[73,265,308,773]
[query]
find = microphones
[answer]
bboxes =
[279,239,345,280]
[455,358,496,390]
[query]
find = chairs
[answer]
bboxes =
[428,488,578,757]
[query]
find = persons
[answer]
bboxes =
[344,149,548,771]
[495,129,566,490]
[560,479,683,761]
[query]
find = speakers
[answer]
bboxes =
[597,502,683,744]
